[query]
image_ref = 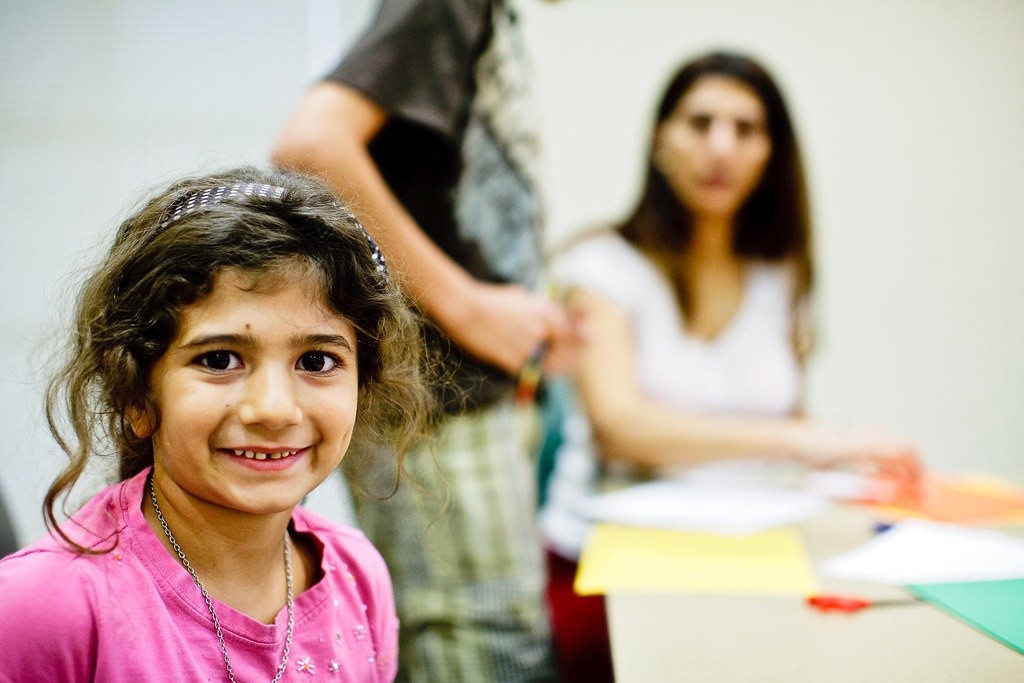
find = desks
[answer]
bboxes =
[593,476,1024,683]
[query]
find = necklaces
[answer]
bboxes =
[148,476,295,683]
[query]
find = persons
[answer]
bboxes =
[537,53,904,683]
[0,164,432,683]
[269,0,582,683]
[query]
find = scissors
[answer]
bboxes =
[808,595,921,612]
